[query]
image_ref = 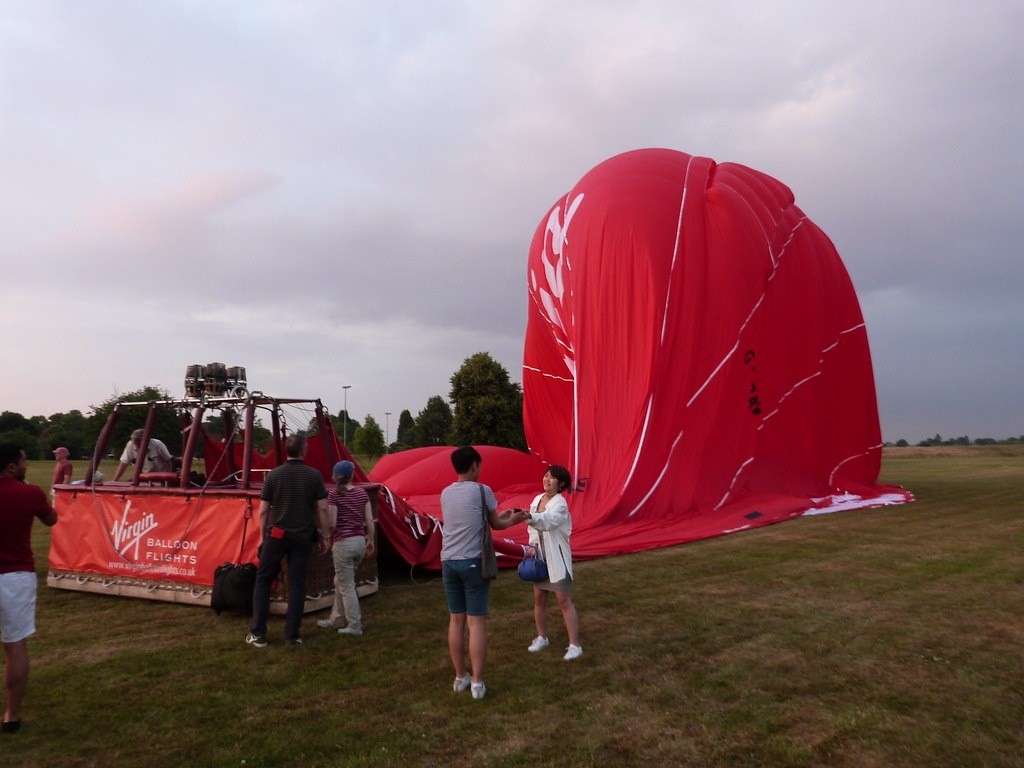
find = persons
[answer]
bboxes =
[46,447,73,507]
[440,446,526,700]
[526,466,582,660]
[245,434,331,647]
[0,440,57,726]
[317,460,374,635]
[114,428,174,486]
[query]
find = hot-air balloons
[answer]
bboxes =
[45,149,917,617]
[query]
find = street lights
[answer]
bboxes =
[342,385,351,448]
[384,413,392,454]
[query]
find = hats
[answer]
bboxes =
[53,447,68,457]
[334,460,354,477]
[131,429,145,438]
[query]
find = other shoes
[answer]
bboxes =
[2,717,22,734]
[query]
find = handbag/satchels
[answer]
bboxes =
[518,546,549,582]
[478,482,498,580]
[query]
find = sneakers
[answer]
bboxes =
[286,639,302,648]
[527,635,549,652]
[470,679,485,699]
[454,672,472,692]
[338,626,362,635]
[317,619,334,627]
[563,644,582,660]
[246,633,267,647]
